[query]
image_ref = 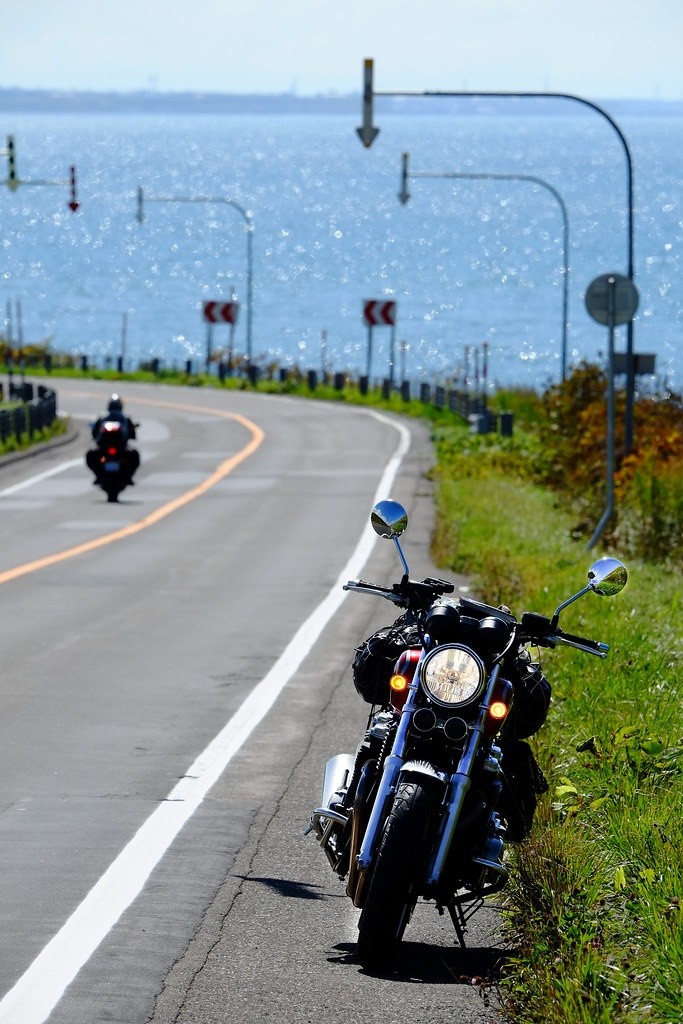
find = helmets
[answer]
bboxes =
[107,398,123,414]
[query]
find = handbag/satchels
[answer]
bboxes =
[352,623,552,742]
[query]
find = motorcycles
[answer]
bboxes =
[301,497,631,982]
[86,420,142,505]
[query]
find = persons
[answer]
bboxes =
[84,396,140,487]
